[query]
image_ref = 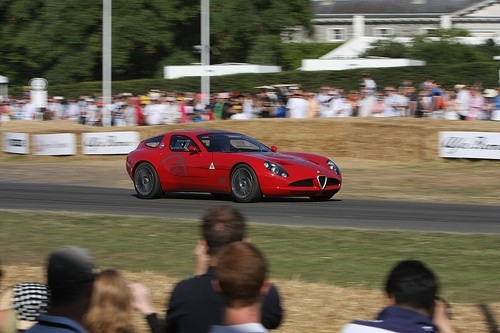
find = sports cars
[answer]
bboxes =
[125,129,343,203]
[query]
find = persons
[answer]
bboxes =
[25,246,97,333]
[162,205,285,333]
[337,257,458,333]
[199,240,275,333]
[85,268,170,333]
[1,75,500,128]
